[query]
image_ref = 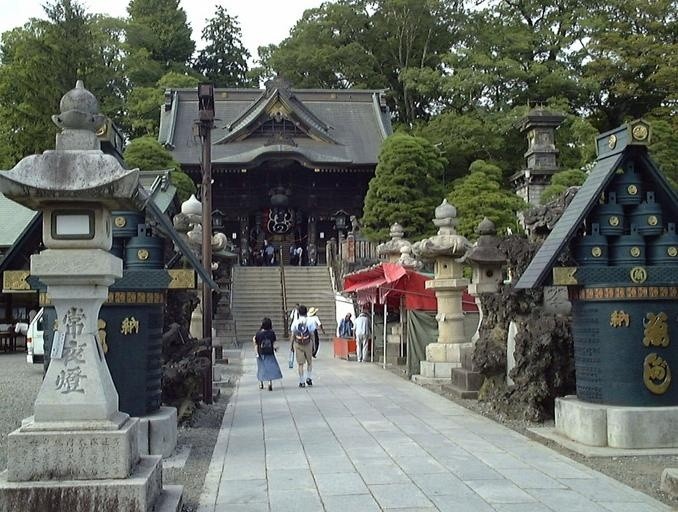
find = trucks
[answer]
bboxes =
[22,305,53,368]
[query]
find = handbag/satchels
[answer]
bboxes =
[289,351,294,368]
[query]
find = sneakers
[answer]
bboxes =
[269,385,272,391]
[306,378,312,385]
[259,384,263,389]
[299,383,305,387]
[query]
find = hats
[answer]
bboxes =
[307,307,319,316]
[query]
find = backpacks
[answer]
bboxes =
[260,332,274,354]
[295,318,310,345]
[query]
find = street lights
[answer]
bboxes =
[522,167,533,205]
[332,207,349,260]
[189,76,221,405]
[210,206,226,239]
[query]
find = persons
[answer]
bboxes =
[290,304,300,323]
[28,307,38,325]
[306,307,326,359]
[352,312,373,363]
[240,242,304,267]
[12,322,29,353]
[336,312,353,337]
[288,305,313,388]
[253,317,283,391]
